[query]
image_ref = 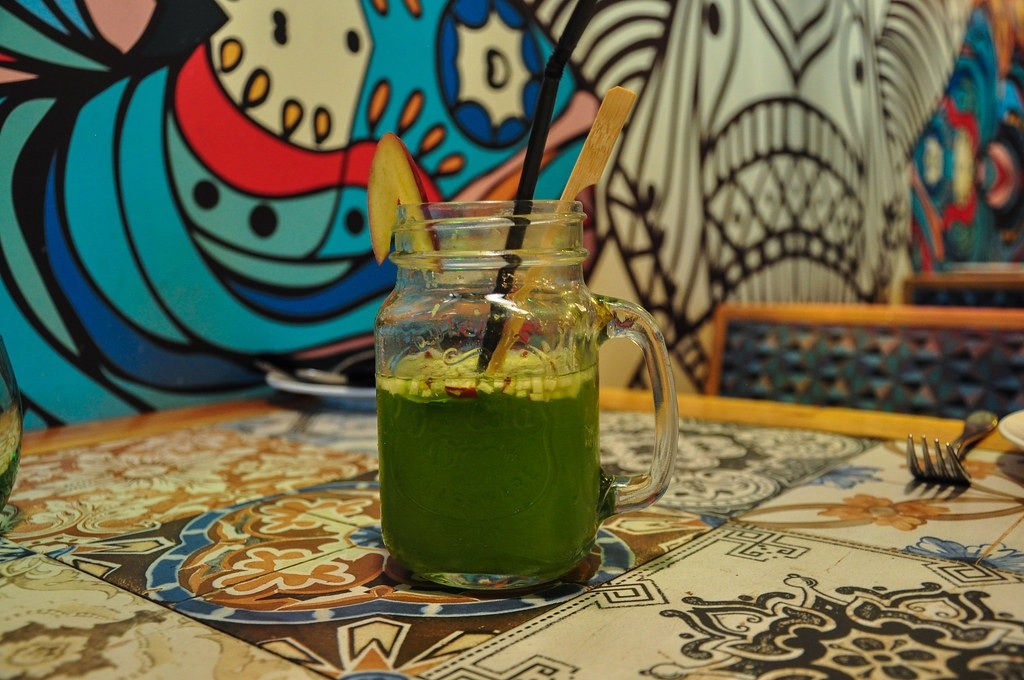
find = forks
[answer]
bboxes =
[906,410,999,487]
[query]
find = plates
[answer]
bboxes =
[997,408,1024,451]
[267,369,378,403]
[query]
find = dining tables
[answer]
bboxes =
[2,377,1022,679]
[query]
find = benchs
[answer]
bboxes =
[900,271,1024,312]
[704,301,1024,428]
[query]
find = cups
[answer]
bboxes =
[372,197,681,591]
